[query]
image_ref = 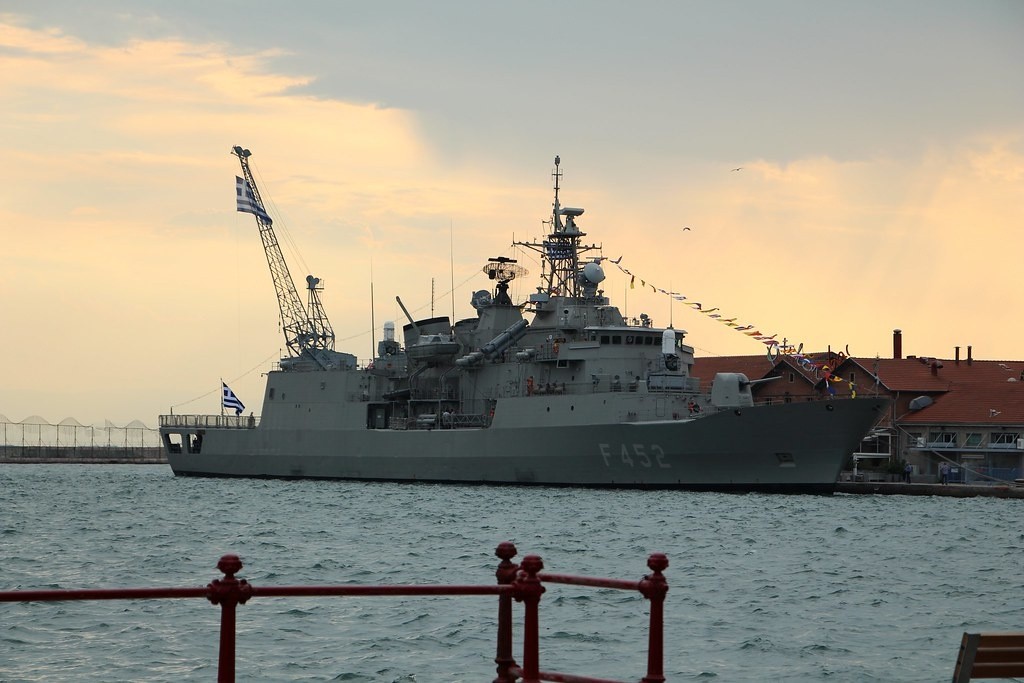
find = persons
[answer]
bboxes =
[941,463,950,485]
[906,462,912,484]
[442,409,454,425]
[688,402,701,414]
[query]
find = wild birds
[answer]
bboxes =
[683,227,690,231]
[732,167,742,171]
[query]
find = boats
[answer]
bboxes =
[156,144,895,492]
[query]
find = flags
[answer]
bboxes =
[235,176,273,225]
[223,382,245,416]
